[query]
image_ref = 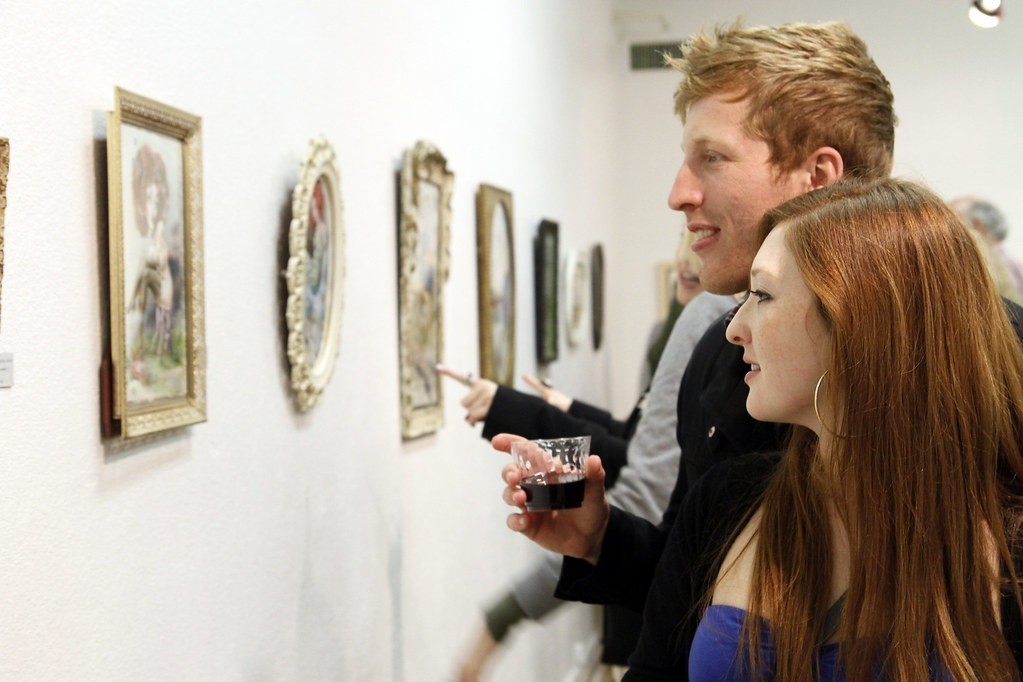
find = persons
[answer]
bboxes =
[435,22,1023,682]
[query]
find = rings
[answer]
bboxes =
[540,376,551,387]
[467,373,473,381]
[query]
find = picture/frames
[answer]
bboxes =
[106,83,208,439]
[284,134,345,416]
[476,182,514,390]
[538,219,559,362]
[400,139,455,440]
[565,251,587,346]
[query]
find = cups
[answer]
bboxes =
[510,435,592,511]
